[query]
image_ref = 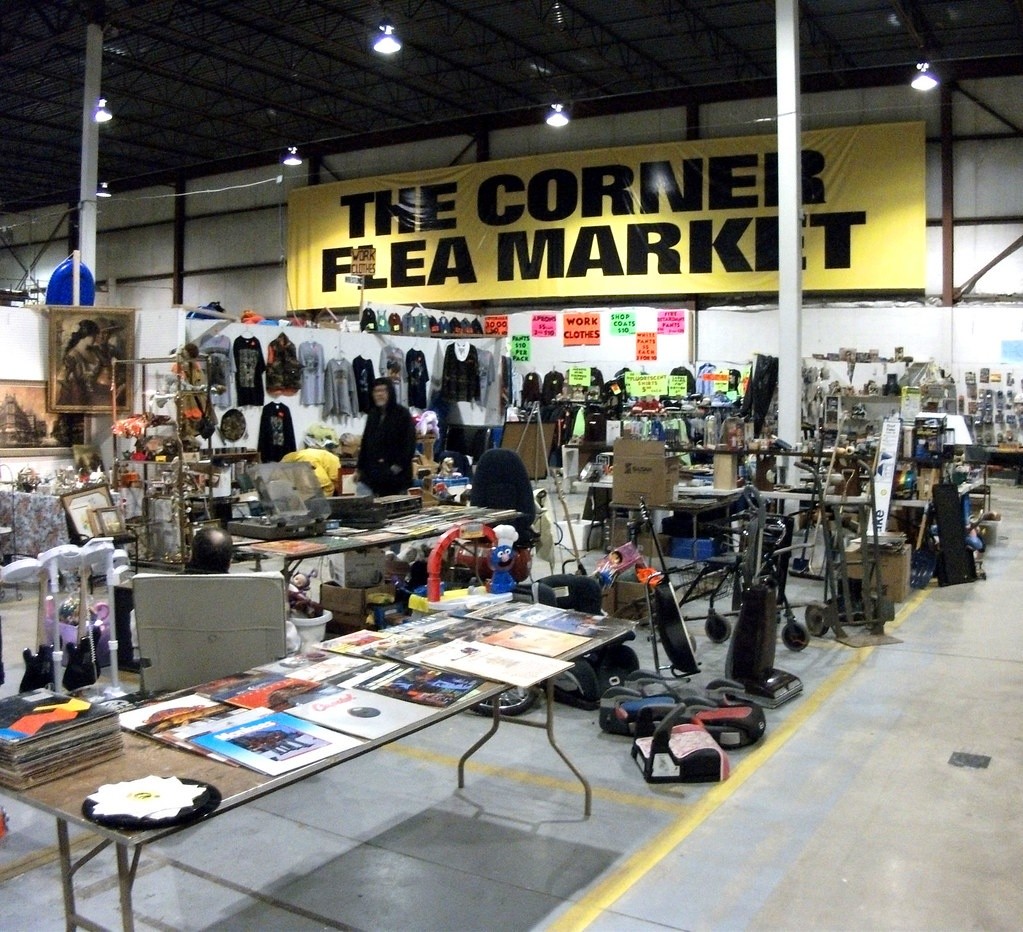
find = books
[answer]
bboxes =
[1,599,640,793]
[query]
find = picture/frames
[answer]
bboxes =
[0,378,91,456]
[46,304,140,415]
[60,486,120,543]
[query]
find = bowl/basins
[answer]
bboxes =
[284,610,333,642]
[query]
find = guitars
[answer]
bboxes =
[20,579,56,694]
[62,564,101,693]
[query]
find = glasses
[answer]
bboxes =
[373,388,388,395]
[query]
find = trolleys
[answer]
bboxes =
[675,512,812,652]
[794,461,904,648]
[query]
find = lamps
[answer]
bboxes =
[99,96,113,121]
[546,97,569,127]
[373,6,401,52]
[96,178,112,198]
[282,140,302,166]
[910,56,938,90]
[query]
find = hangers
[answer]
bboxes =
[183,303,713,403]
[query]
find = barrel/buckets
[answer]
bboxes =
[365,592,399,630]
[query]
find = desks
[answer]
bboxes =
[1,598,640,932]
[958,444,1023,478]
[240,509,528,612]
[610,496,735,592]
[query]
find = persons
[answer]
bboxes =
[352,378,416,554]
[56,317,127,406]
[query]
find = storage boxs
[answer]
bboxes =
[413,434,470,489]
[319,549,394,629]
[601,579,653,621]
[843,465,998,602]
[557,440,727,560]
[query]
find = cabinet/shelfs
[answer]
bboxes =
[114,351,216,569]
[619,419,948,558]
